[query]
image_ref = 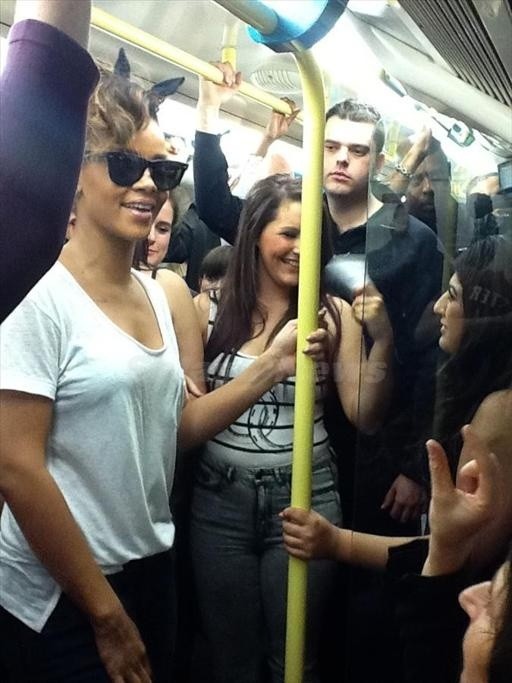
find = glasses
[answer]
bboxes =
[87,152,188,190]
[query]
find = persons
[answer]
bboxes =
[0,0,512,683]
